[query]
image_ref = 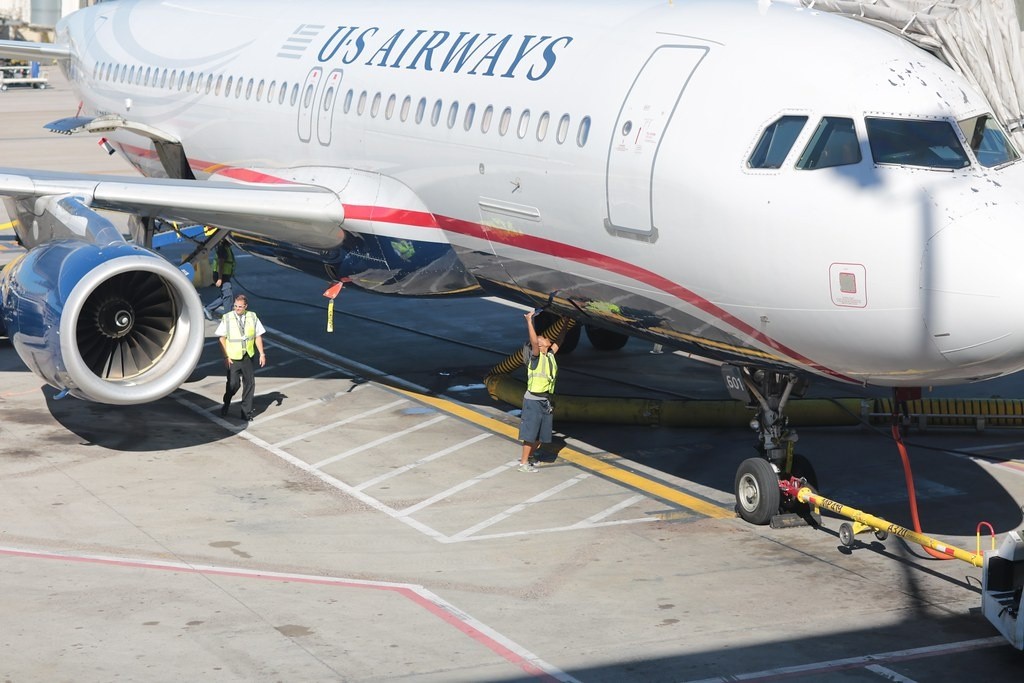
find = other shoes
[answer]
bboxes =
[221,401,230,417]
[529,456,543,466]
[518,463,538,473]
[204,307,213,320]
[245,417,252,421]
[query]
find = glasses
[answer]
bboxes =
[233,303,246,309]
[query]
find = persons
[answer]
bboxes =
[213,294,267,420]
[517,309,569,473]
[551,289,667,330]
[390,237,431,267]
[204,240,235,320]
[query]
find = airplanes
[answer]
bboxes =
[0,1,1023,524]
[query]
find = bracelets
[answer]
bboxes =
[225,356,229,359]
[260,353,265,356]
